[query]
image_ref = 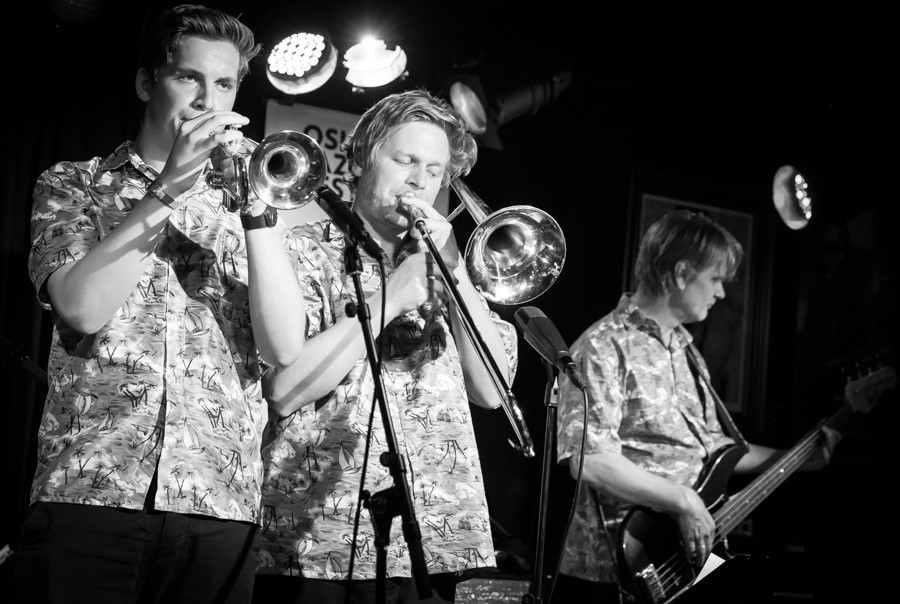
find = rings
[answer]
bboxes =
[697,545,707,550]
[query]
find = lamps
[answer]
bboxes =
[252,13,407,96]
[771,155,865,230]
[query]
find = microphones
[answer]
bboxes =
[312,183,392,263]
[512,305,591,392]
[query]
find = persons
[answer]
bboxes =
[253,90,521,604]
[558,210,837,604]
[20,4,312,604]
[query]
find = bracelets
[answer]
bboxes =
[146,181,178,209]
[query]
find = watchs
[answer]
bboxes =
[239,203,279,231]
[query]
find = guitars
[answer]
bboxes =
[615,362,900,604]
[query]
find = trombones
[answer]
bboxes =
[400,172,570,460]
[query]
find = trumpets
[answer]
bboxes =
[206,124,329,215]
